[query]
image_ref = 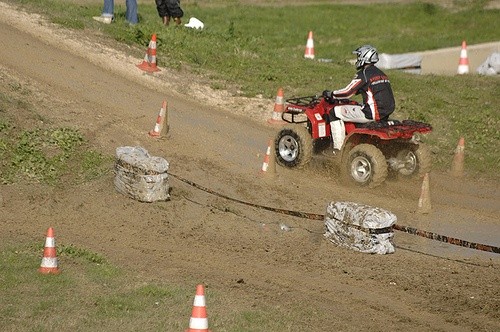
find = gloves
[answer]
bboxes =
[322,90,333,99]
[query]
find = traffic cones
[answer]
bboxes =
[259,140,270,173]
[450,138,466,176]
[137,32,160,73]
[303,31,315,59]
[453,41,470,76]
[147,101,170,138]
[182,283,209,332]
[38,227,61,275]
[418,172,431,211]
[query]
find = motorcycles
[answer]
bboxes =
[274,91,432,191]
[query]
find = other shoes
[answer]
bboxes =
[92,16,111,25]
[321,147,341,158]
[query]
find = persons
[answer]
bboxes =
[155,0,184,25]
[92,0,138,25]
[321,45,395,158]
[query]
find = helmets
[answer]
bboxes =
[352,45,379,70]
